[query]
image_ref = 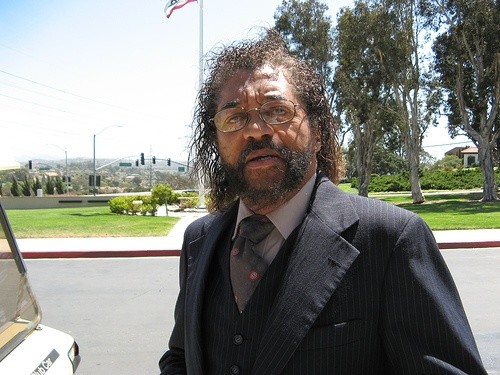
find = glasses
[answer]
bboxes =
[207,100,308,132]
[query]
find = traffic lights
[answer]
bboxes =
[167,158,170,166]
[135,159,139,166]
[152,156,155,164]
[141,153,145,165]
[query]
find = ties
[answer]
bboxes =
[230,217,274,311]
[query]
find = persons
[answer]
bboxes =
[158,30,489,375]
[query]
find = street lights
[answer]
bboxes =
[93,124,124,195]
